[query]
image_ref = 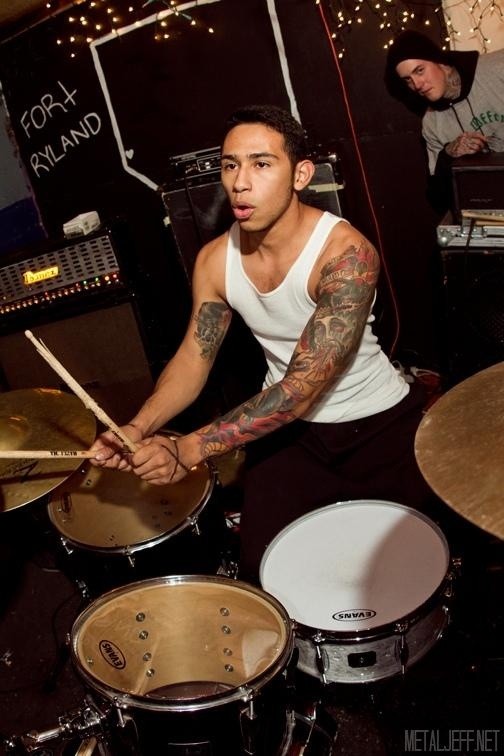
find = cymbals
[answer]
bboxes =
[414,360,504,539]
[0,389,98,514]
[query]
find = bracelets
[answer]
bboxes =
[125,421,146,439]
[160,436,193,481]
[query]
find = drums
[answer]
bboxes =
[47,429,238,603]
[259,501,463,686]
[63,573,297,756]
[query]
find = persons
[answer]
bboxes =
[84,102,416,582]
[387,31,504,203]
